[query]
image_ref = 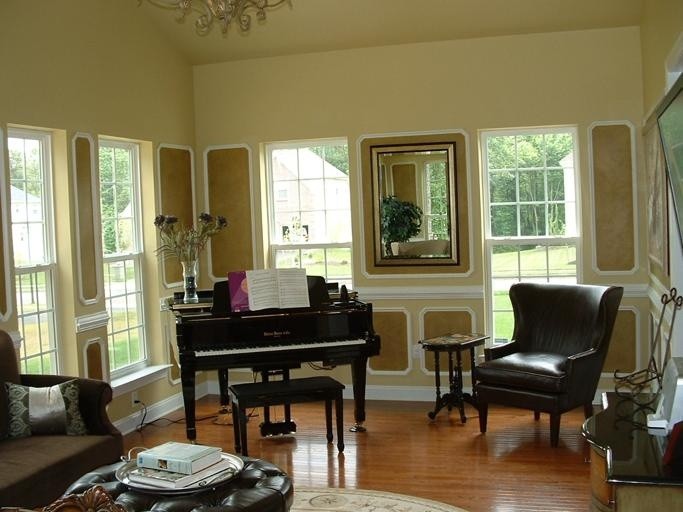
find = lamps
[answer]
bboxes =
[136,0,293,37]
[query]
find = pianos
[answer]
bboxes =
[168,277,381,439]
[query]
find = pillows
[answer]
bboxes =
[3,378,86,439]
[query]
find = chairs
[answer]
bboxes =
[473,282,623,450]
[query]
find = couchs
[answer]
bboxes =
[0,330,123,512]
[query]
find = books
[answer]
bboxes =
[245,268,311,311]
[226,271,249,313]
[128,441,231,488]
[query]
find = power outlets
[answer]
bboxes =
[132,393,141,407]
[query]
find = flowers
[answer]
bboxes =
[154,212,228,262]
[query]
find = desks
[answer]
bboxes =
[581,391,683,512]
[61,453,293,512]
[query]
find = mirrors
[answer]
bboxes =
[370,141,458,268]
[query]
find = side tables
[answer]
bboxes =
[420,331,491,424]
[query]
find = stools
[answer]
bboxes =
[228,376,345,456]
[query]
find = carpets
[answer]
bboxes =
[290,487,469,512]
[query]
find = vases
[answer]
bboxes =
[181,261,199,304]
